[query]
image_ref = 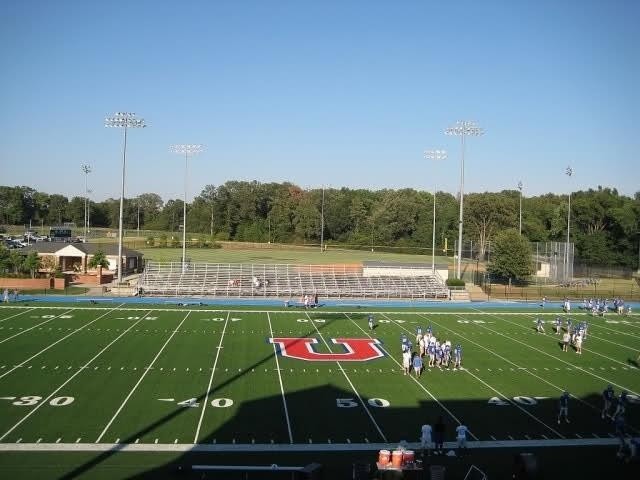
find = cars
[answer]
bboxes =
[0,231,49,248]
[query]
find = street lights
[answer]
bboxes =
[565,165,575,280]
[79,159,97,244]
[443,118,488,281]
[103,111,153,283]
[423,148,448,276]
[517,179,528,235]
[168,143,209,275]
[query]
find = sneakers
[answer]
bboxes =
[566,419,570,423]
[557,420,561,424]
[601,414,616,421]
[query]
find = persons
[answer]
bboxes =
[536,295,633,354]
[185,261,190,271]
[139,287,144,298]
[433,415,446,456]
[419,417,433,457]
[601,385,640,465]
[304,294,308,308]
[455,422,469,449]
[229,276,269,287]
[401,326,463,378]
[557,392,571,424]
[4,288,19,304]
[314,291,318,308]
[368,314,375,330]
